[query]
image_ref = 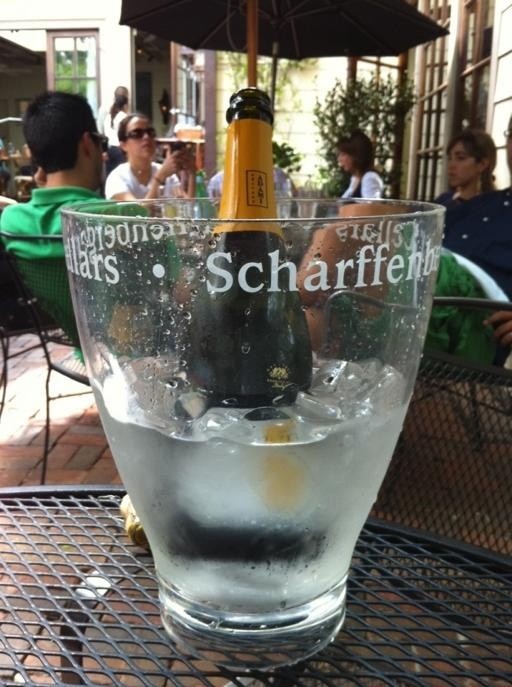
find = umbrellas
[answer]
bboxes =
[116,0,450,92]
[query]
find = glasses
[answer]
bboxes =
[126,126,157,140]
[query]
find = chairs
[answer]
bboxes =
[1,267,65,420]
[317,285,509,646]
[4,229,178,486]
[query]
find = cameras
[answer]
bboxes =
[163,140,186,158]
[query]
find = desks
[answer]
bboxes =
[0,483,512,687]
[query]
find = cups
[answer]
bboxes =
[53,195,449,675]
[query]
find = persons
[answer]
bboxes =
[105,114,289,218]
[104,86,130,166]
[0,91,191,390]
[334,133,383,203]
[408,129,497,230]
[1,138,11,194]
[295,115,511,367]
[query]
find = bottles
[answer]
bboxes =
[170,83,321,410]
[191,168,215,220]
